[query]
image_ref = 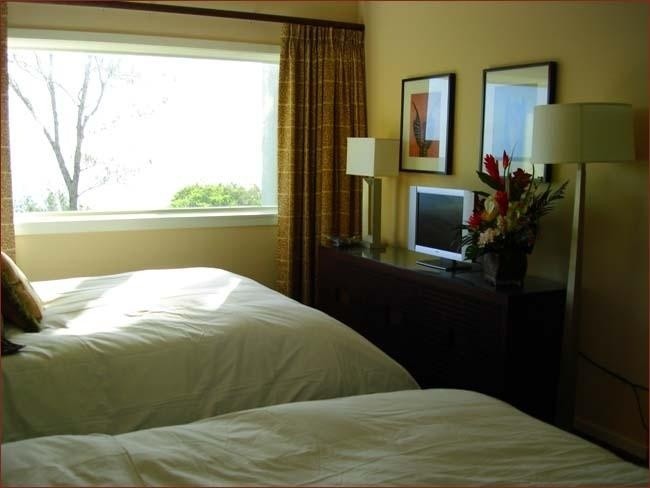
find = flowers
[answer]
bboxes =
[456,144,569,261]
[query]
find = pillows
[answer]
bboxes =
[1,250,43,333]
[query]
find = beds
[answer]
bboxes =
[1,387,650,488]
[1,267,421,445]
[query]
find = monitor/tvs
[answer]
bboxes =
[407,185,476,270]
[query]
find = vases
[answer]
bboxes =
[484,250,528,291]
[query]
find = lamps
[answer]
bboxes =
[346,137,400,249]
[532,102,636,434]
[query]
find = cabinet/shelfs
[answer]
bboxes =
[318,238,566,425]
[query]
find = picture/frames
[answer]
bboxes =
[479,61,556,184]
[398,73,455,175]
